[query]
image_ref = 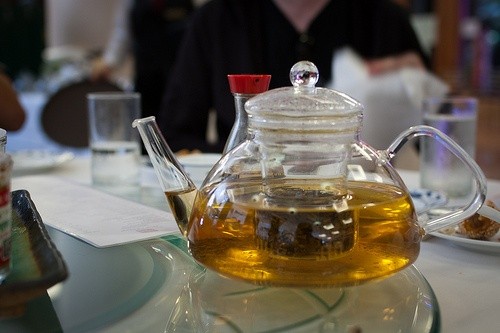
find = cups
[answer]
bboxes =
[0,154,11,282]
[421,97,477,208]
[87,92,140,190]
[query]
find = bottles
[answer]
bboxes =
[221,74,273,203]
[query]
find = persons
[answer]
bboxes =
[160,1,429,155]
[88,0,208,121]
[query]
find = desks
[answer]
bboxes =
[0,149,499,333]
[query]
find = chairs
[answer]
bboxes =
[41,79,126,148]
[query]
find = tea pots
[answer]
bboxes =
[133,61,487,290]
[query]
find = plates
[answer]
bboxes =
[419,215,500,254]
[0,190,66,307]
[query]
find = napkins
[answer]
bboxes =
[333,46,452,150]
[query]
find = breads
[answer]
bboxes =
[457,212,500,239]
